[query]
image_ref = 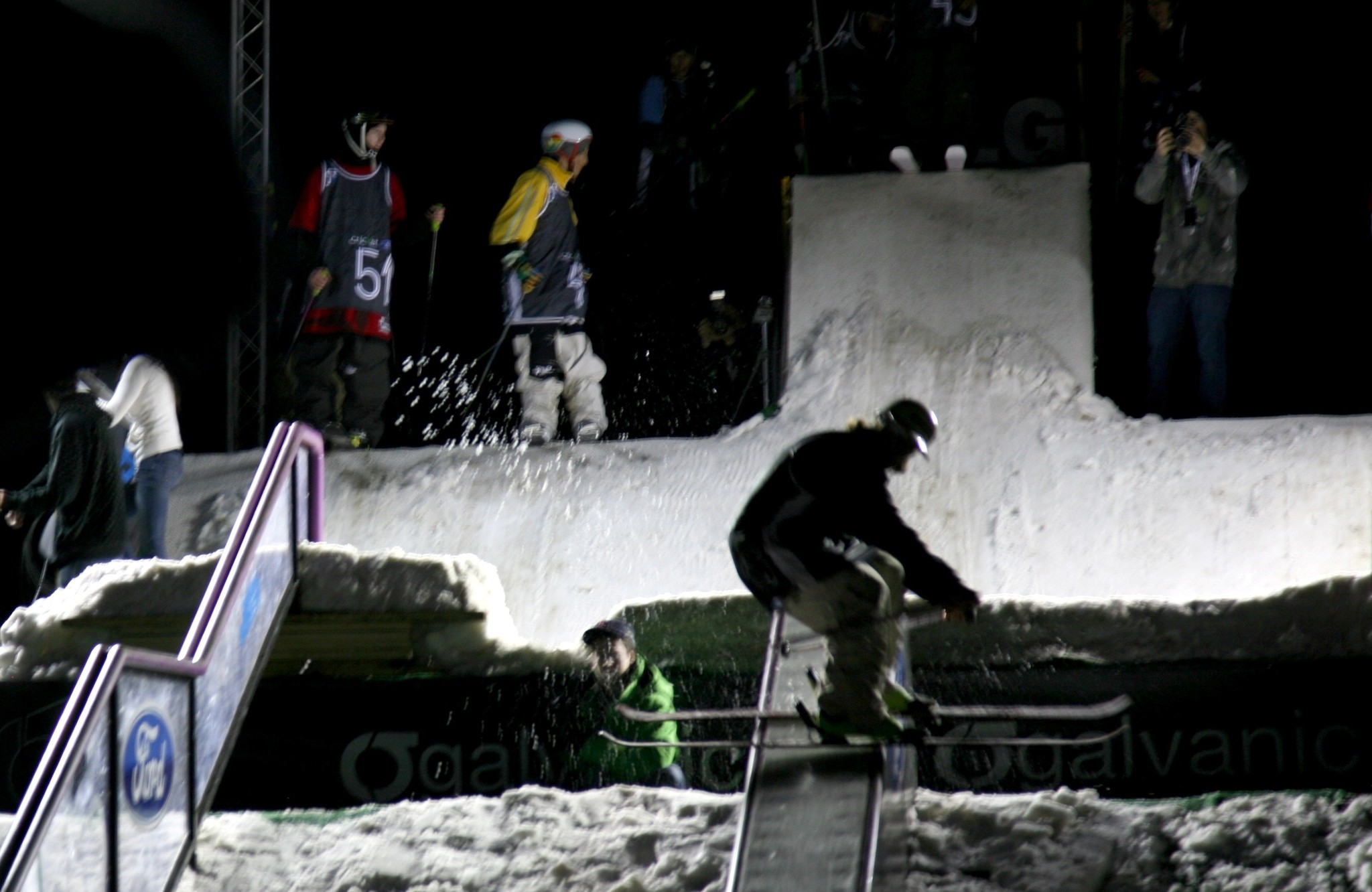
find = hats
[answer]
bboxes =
[582,619,636,652]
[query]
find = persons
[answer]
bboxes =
[0,375,128,585]
[730,399,982,741]
[639,47,718,191]
[76,331,182,559]
[121,443,143,518]
[583,621,681,789]
[289,103,445,451]
[490,121,608,442]
[1136,106,1248,419]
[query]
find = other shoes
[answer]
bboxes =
[880,669,940,724]
[518,423,553,444]
[573,420,600,443]
[819,694,904,746]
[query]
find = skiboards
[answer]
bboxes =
[506,428,636,446]
[597,696,1133,748]
[887,145,968,172]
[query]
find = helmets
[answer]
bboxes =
[880,399,938,461]
[541,120,593,156]
[334,107,387,156]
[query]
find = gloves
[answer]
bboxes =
[939,587,980,625]
